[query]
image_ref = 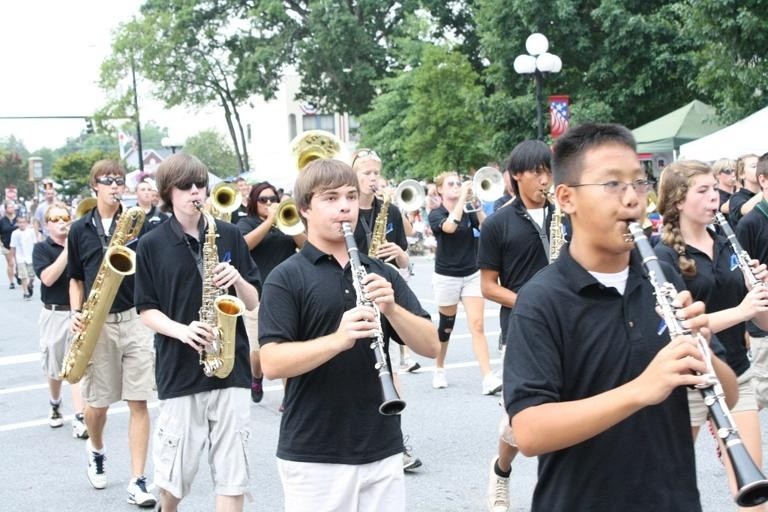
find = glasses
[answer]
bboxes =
[567,178,656,195]
[48,215,71,223]
[96,176,124,186]
[448,181,462,187]
[256,196,279,203]
[174,177,207,190]
[720,168,735,175]
[351,149,378,168]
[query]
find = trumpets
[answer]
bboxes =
[206,182,242,221]
[463,167,504,214]
[60,197,96,235]
[395,179,425,212]
[276,200,305,236]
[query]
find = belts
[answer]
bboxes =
[44,304,70,311]
[104,306,137,323]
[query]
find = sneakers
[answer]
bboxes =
[9,274,34,300]
[431,368,448,389]
[403,446,423,469]
[486,455,511,512]
[481,376,502,395]
[51,397,158,507]
[399,358,421,372]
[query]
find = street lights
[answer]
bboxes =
[512,31,561,142]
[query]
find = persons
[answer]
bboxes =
[1,123,767,511]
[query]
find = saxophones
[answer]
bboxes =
[61,194,145,384]
[192,201,244,378]
[542,191,567,264]
[368,184,391,260]
